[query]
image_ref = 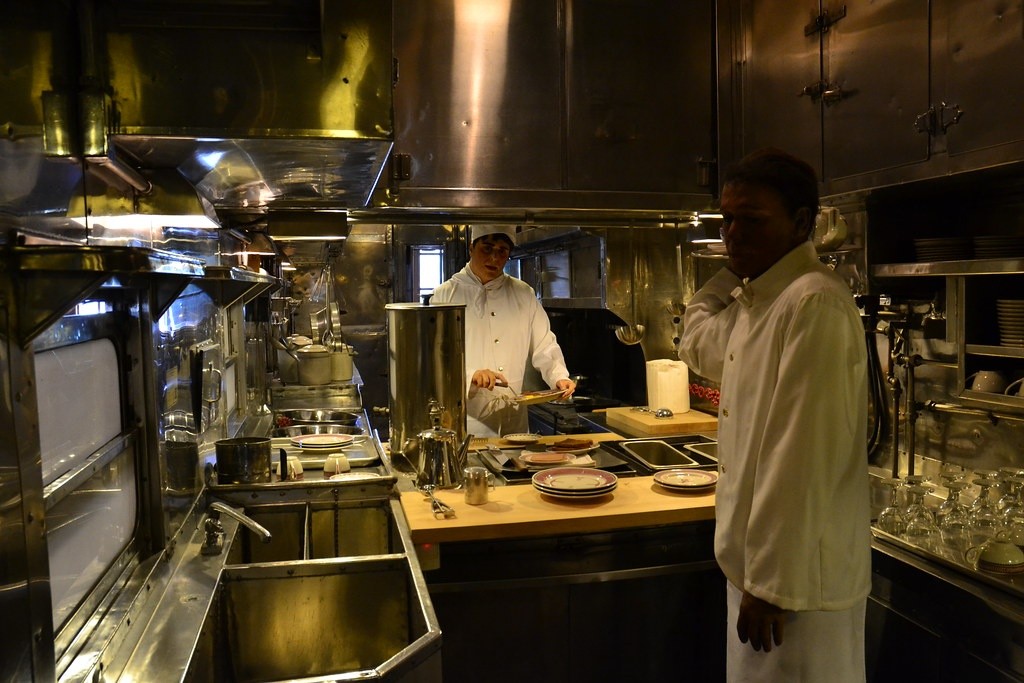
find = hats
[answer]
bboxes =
[470,225,517,248]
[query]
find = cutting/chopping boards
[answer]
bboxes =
[605,405,719,435]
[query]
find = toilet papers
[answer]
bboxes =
[646,357,689,415]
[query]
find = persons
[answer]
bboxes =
[677,150,873,683]
[428,223,576,439]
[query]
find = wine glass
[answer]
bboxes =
[876,466,1024,563]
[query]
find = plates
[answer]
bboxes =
[997,299,1024,348]
[653,469,718,491]
[502,433,543,443]
[545,442,600,455]
[329,471,381,480]
[531,466,622,500]
[507,389,567,405]
[291,433,356,454]
[519,452,576,464]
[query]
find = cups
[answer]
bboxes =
[323,453,351,480]
[275,456,304,482]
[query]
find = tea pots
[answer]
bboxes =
[403,417,475,490]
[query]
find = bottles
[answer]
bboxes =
[971,370,1004,393]
[277,333,354,385]
[464,466,496,505]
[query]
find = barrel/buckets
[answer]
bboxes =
[213,436,272,484]
[382,293,469,473]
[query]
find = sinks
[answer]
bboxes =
[182,555,432,683]
[221,499,408,568]
[688,442,717,461]
[621,438,695,469]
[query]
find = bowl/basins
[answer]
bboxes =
[975,539,1024,575]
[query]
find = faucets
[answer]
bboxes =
[204,501,272,558]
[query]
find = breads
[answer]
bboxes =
[553,438,593,450]
